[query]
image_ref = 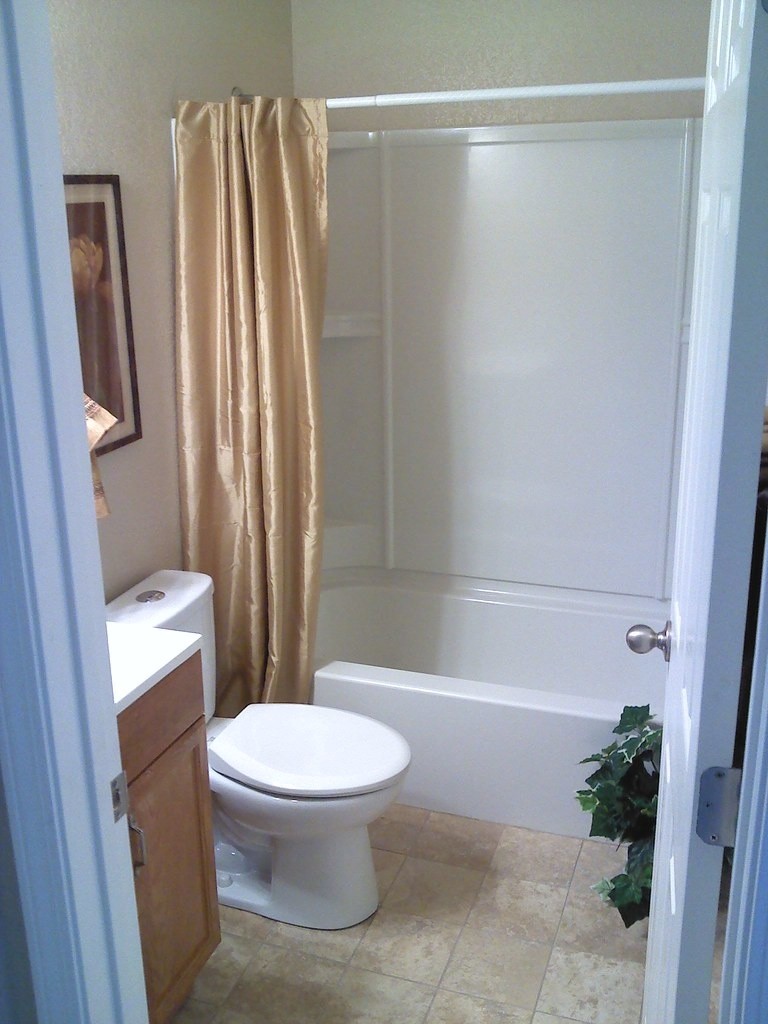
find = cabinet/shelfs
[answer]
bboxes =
[104,619,224,1023]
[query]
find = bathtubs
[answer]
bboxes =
[311,573,671,850]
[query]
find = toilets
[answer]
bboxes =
[104,569,414,932]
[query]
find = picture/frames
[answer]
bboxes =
[62,175,146,467]
